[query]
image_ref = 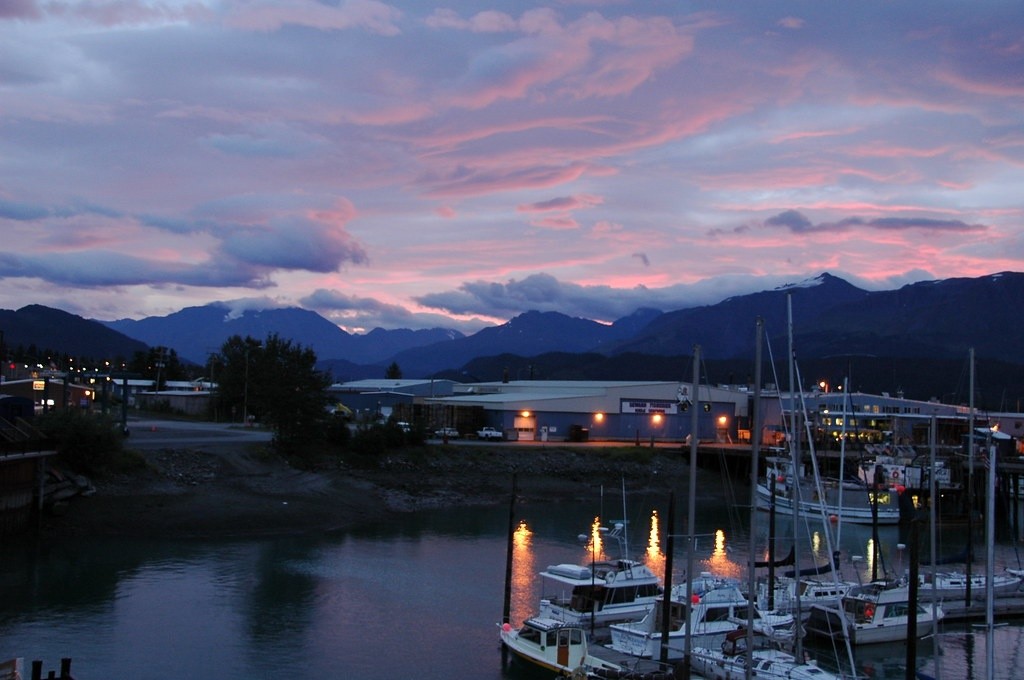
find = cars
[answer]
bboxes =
[396,421,412,434]
[423,427,433,439]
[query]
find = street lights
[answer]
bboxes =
[243,345,264,424]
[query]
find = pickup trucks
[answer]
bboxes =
[476,426,503,442]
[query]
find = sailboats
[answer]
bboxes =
[493,287,1024,680]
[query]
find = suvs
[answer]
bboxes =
[435,427,460,440]
